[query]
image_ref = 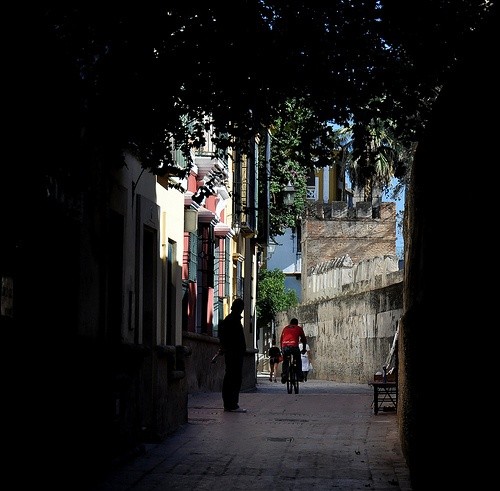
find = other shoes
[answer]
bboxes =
[269,378,277,383]
[223,406,248,413]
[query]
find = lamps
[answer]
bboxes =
[270,179,298,213]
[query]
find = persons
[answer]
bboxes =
[210,298,248,413]
[269,318,313,394]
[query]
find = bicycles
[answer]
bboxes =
[280,350,307,394]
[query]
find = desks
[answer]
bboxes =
[372,374,398,416]
